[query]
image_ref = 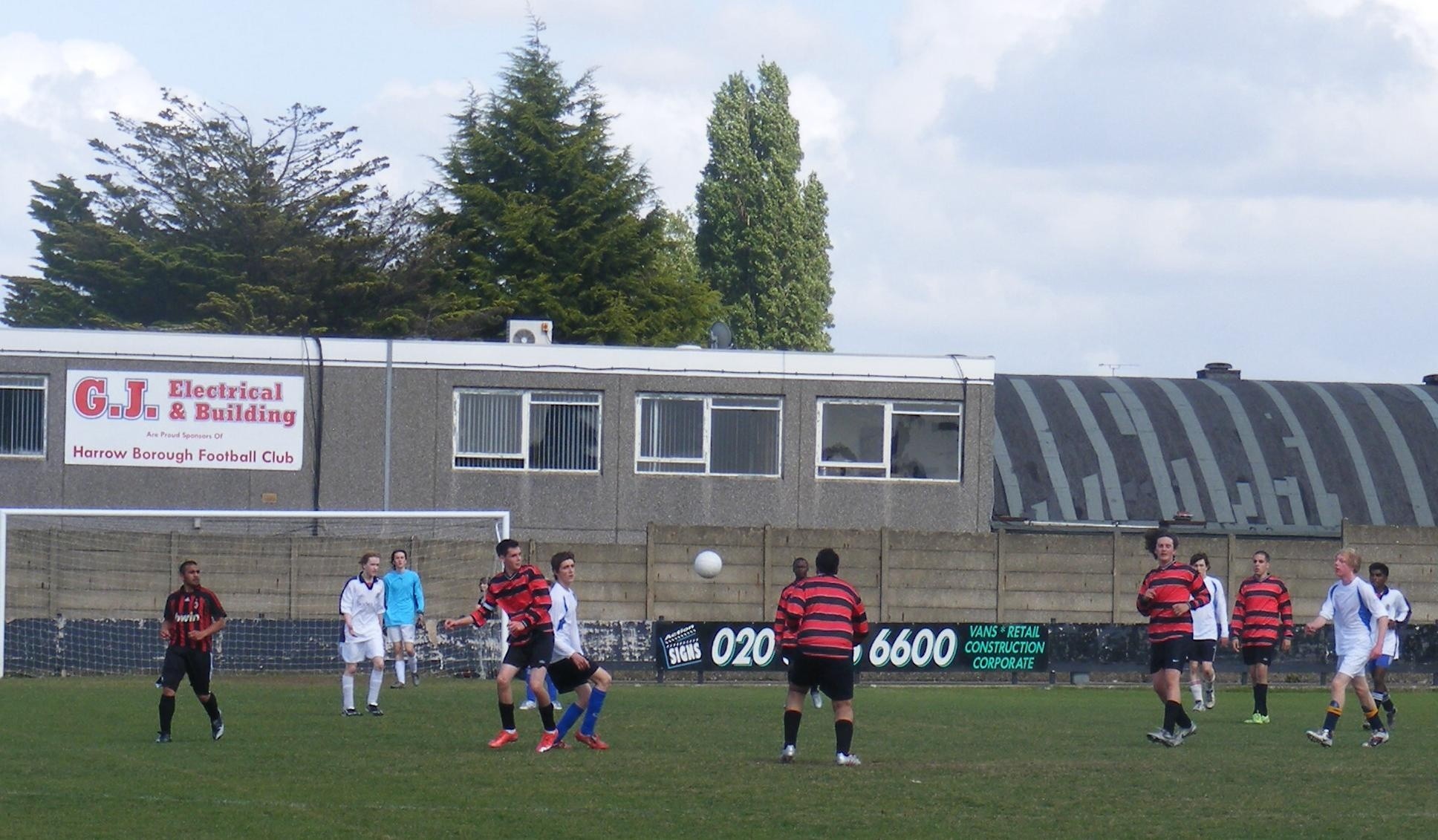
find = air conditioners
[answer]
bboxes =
[506,319,553,345]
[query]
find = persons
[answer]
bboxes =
[1362,563,1412,729]
[548,551,612,750]
[445,539,559,754]
[476,577,564,710]
[154,560,224,742]
[1305,547,1388,749]
[1136,533,1211,748]
[782,548,868,765]
[338,553,387,717]
[1188,553,1229,711]
[383,550,424,688]
[774,558,822,710]
[1232,551,1294,721]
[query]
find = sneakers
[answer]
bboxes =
[535,728,559,753]
[1363,719,1371,732]
[519,700,537,711]
[365,703,384,715]
[341,709,363,716]
[1387,707,1397,730]
[1306,728,1333,748]
[574,728,609,749]
[1173,720,1197,746]
[780,745,796,762]
[489,728,519,748]
[1146,726,1175,747]
[411,671,420,687]
[1205,687,1216,708]
[1245,711,1270,724]
[810,690,822,709]
[836,752,862,766]
[391,682,404,689]
[210,709,225,741]
[1193,701,1206,712]
[1361,731,1390,748]
[155,730,172,744]
[552,740,570,749]
[552,700,563,709]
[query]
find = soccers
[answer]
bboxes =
[694,550,723,579]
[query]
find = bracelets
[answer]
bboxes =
[1283,637,1292,640]
[1231,636,1237,640]
